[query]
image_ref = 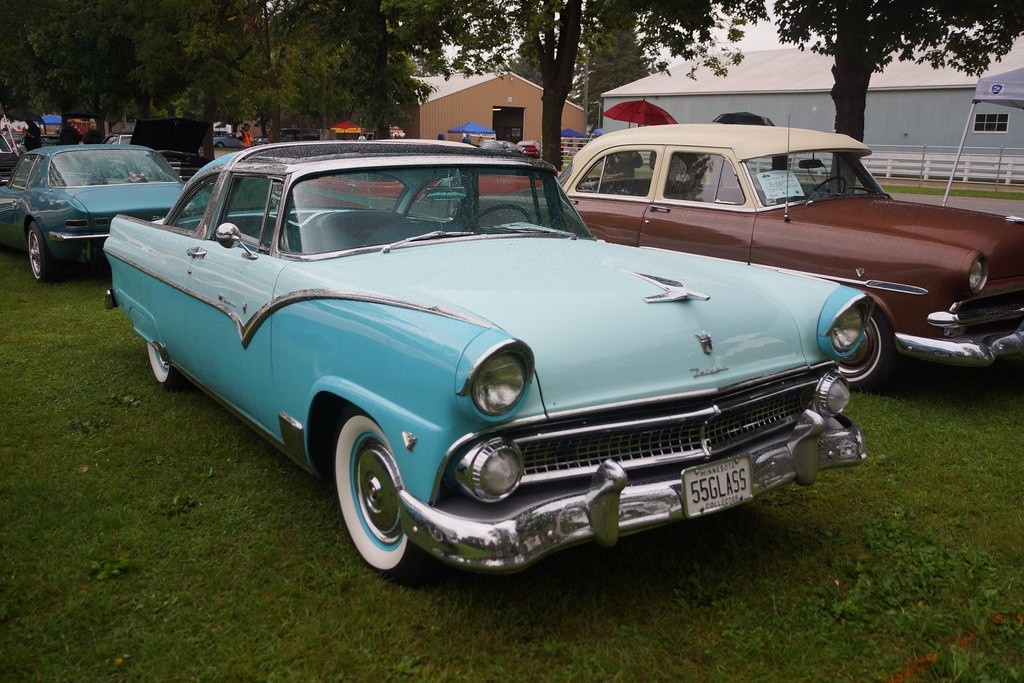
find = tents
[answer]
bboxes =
[941,68,1024,207]
[446,123,496,146]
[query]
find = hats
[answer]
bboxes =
[244,123,251,127]
[437,133,445,140]
[357,135,366,140]
[67,118,74,122]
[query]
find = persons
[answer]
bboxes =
[606,151,645,179]
[22,119,41,153]
[462,135,471,144]
[84,122,103,143]
[60,119,82,145]
[236,122,254,153]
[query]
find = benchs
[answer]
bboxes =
[592,178,650,197]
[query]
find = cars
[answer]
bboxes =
[280,128,320,142]
[212,131,241,148]
[19,134,62,152]
[497,123,1024,392]
[0,144,185,283]
[293,171,564,214]
[101,118,215,183]
[479,140,523,156]
[0,133,20,186]
[516,140,543,159]
[98,139,877,589]
[261,138,269,144]
[4,130,26,149]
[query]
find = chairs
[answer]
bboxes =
[284,209,421,260]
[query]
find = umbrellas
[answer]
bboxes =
[33,115,62,125]
[327,121,366,140]
[601,97,680,130]
[560,128,584,139]
[714,112,775,127]
